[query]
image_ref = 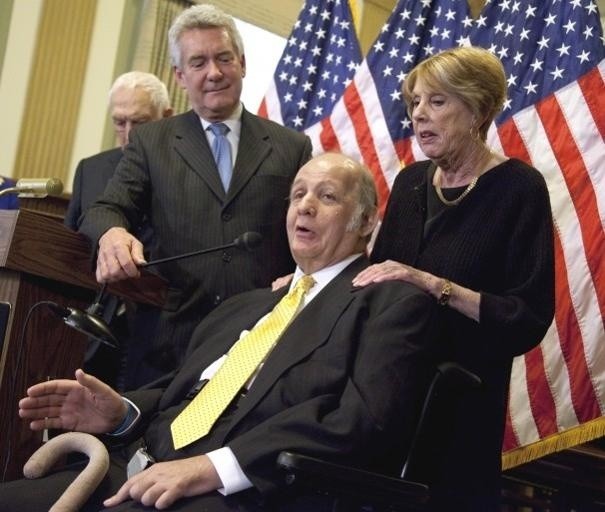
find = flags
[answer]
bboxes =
[459,2,604,473]
[252,1,360,145]
[311,0,473,251]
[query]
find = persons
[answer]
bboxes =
[2,150,429,510]
[351,46,557,510]
[74,6,312,442]
[63,71,176,466]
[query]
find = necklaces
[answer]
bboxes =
[433,150,494,206]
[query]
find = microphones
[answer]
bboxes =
[14,178,66,199]
[63,229,265,346]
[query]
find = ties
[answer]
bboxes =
[167,274,327,453]
[205,120,235,193]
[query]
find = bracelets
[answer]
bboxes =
[437,276,452,306]
